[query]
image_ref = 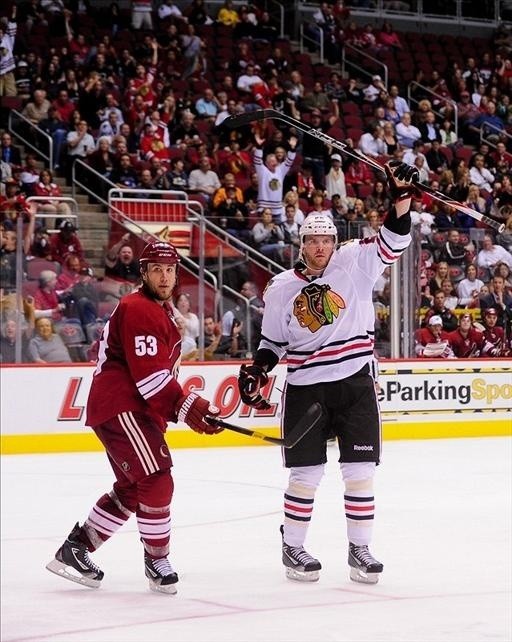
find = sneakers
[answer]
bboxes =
[143,552,181,585]
[279,544,323,571]
[348,543,384,571]
[56,538,106,582]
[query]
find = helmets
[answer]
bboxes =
[299,212,338,247]
[459,313,474,323]
[481,306,498,318]
[140,241,181,278]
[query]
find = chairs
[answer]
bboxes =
[172,0,512,221]
[0,253,124,365]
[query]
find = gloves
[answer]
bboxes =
[239,364,271,410]
[384,159,421,202]
[173,393,226,435]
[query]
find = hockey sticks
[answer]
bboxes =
[204,401,324,449]
[211,106,506,240]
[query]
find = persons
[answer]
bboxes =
[47,240,227,590]
[238,159,423,566]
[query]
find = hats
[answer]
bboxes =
[429,315,442,326]
